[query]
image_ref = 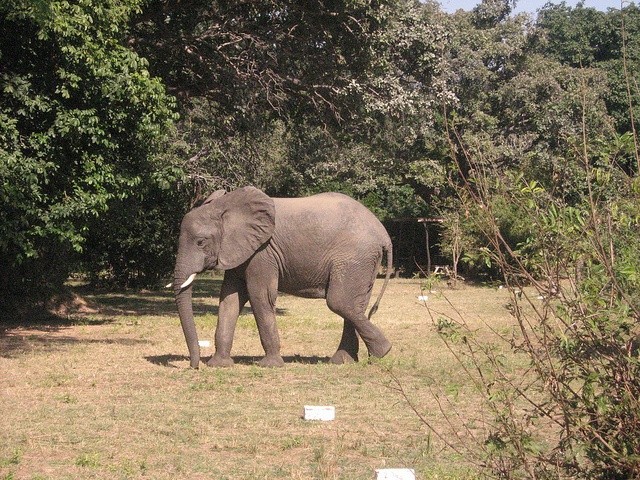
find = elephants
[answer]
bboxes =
[163,184,394,370]
